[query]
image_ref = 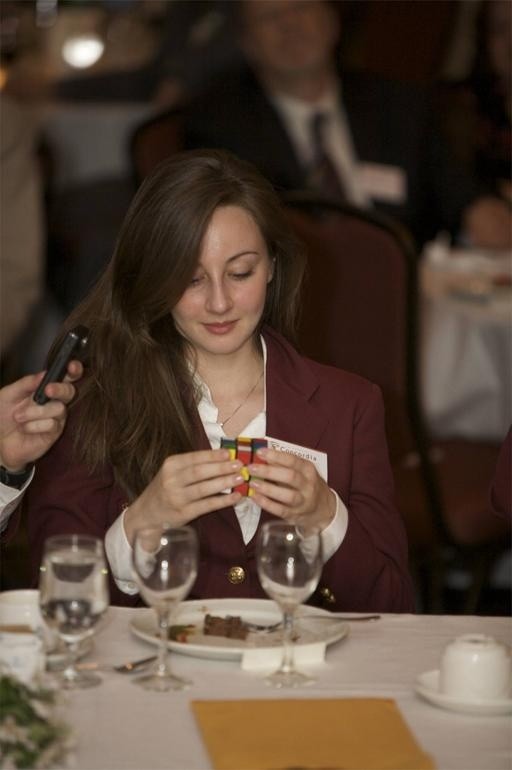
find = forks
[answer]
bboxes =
[238,600,380,639]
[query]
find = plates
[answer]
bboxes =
[415,640,511,713]
[131,576,349,666]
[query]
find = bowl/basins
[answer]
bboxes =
[441,636,506,696]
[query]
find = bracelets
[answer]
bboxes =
[0,464,35,490]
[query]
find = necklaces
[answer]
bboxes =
[220,369,265,430]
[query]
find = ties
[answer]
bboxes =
[306,110,349,206]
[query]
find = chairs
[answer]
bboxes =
[258,189,470,609]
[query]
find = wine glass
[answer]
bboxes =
[261,524,324,688]
[133,524,198,696]
[38,538,101,690]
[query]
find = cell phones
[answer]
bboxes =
[33,324,93,405]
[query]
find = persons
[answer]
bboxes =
[0,358,86,531]
[19,146,417,615]
